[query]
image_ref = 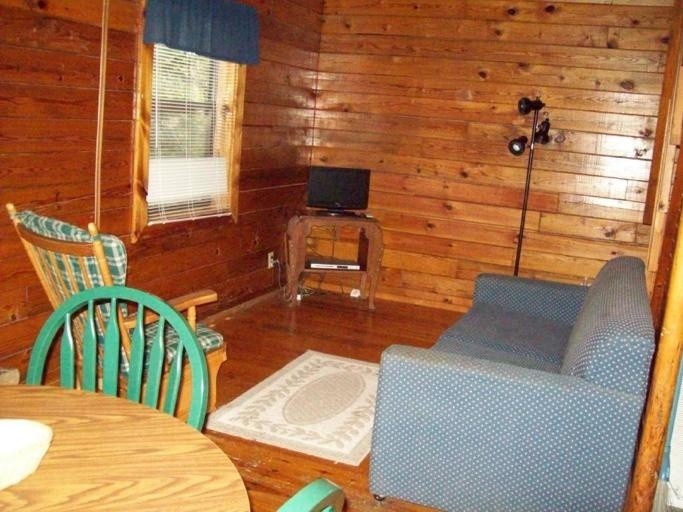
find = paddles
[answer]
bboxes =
[203,349,381,468]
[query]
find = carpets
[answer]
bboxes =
[203,349,381,468]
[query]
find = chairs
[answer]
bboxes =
[6,204,226,423]
[24,285,210,427]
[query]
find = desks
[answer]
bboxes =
[285,211,383,310]
[0,383,250,511]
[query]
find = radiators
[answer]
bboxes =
[363,212,374,219]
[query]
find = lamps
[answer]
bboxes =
[506,98,551,278]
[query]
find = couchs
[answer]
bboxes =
[366,254,656,511]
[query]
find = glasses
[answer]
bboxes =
[506,98,551,278]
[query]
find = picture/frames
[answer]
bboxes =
[285,211,383,310]
[0,383,250,511]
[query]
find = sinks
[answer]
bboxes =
[366,254,656,511]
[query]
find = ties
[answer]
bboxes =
[306,165,371,216]
